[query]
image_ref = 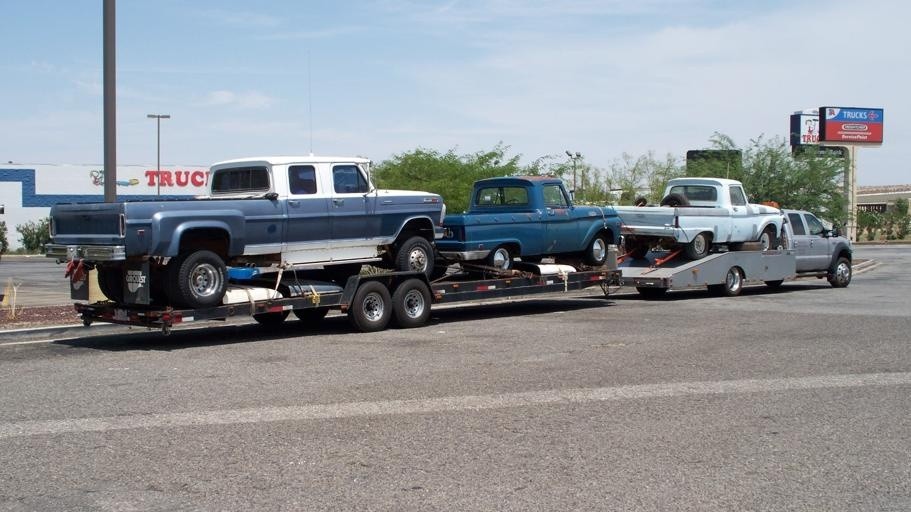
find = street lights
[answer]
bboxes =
[565,151,581,192]
[147,114,171,199]
